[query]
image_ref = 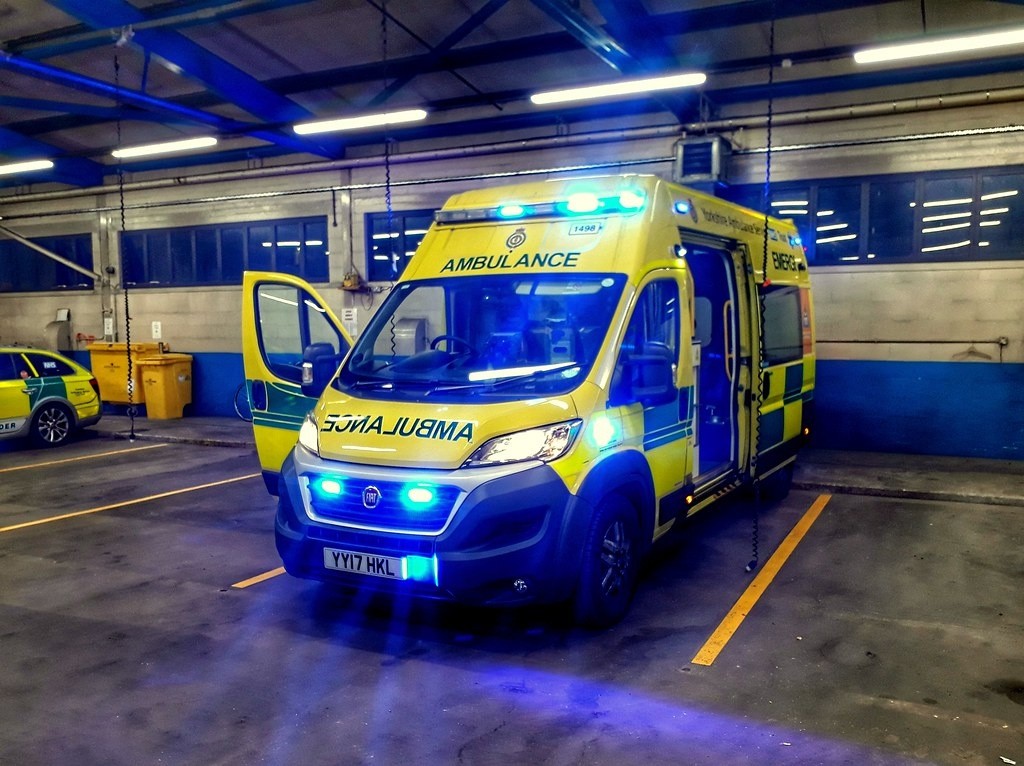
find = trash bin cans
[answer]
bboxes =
[85,341,170,417]
[136,353,194,420]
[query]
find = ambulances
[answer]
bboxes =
[241,173,820,631]
[0,342,103,447]
